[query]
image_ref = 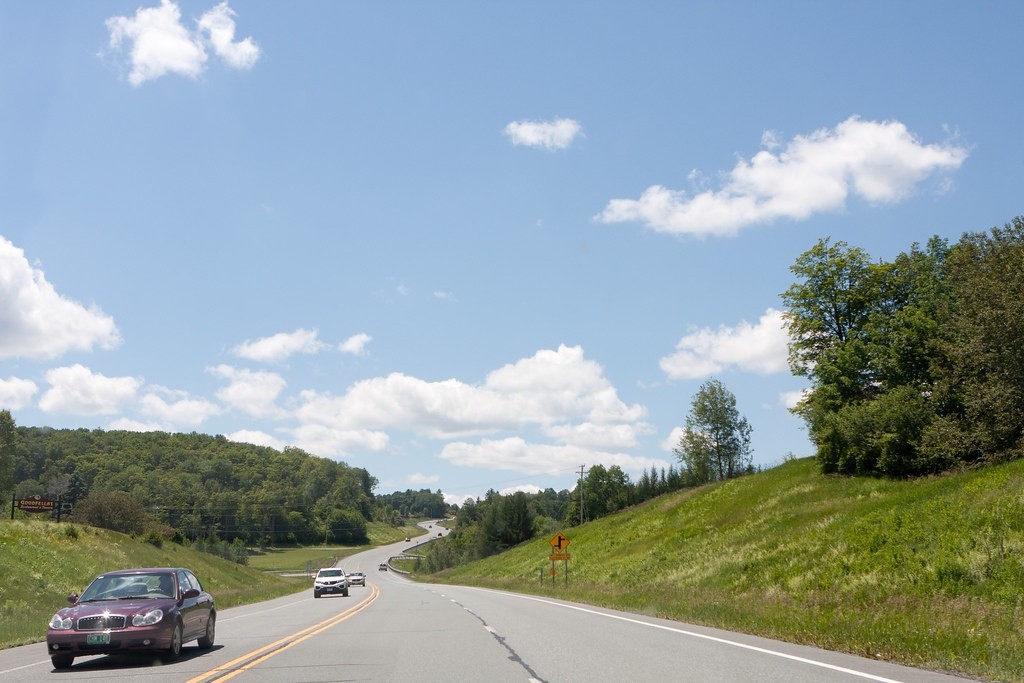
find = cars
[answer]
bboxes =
[47,567,216,670]
[346,572,366,587]
[405,537,410,542]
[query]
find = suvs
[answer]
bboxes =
[378,563,387,571]
[312,568,350,598]
